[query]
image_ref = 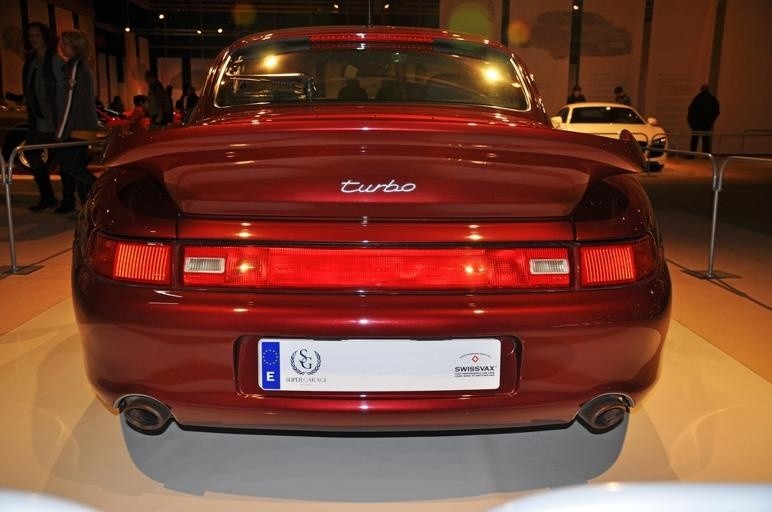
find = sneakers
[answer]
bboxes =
[29,198,59,212]
[53,198,76,215]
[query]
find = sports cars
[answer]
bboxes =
[548,99,673,172]
[70,18,677,454]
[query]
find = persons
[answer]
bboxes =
[110,82,202,127]
[21,22,76,215]
[567,86,586,105]
[686,83,721,160]
[54,27,103,216]
[613,85,631,107]
[143,70,173,128]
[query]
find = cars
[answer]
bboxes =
[0,87,136,176]
